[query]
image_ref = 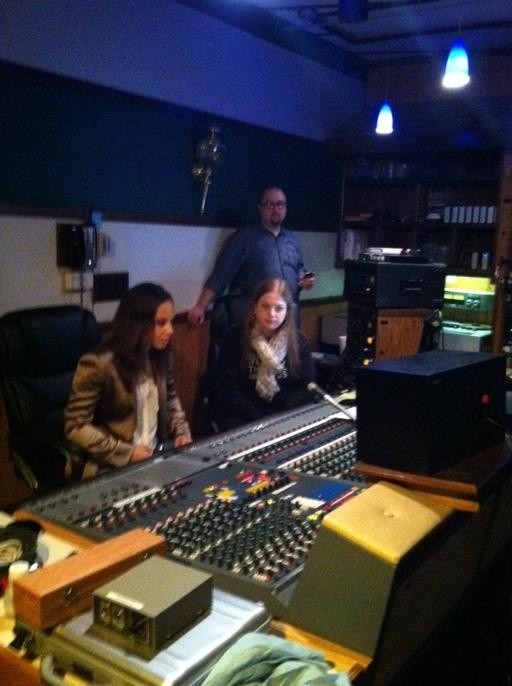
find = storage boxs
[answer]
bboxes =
[343,259,446,310]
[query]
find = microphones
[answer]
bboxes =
[303,379,356,424]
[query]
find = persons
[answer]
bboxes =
[211,277,316,415]
[188,188,314,339]
[64,282,192,477]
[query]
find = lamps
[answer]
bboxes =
[376,65,393,135]
[442,0,470,88]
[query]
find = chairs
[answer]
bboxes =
[192,294,301,440]
[0,305,100,498]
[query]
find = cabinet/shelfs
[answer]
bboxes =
[335,151,505,278]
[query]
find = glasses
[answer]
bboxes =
[261,200,288,209]
